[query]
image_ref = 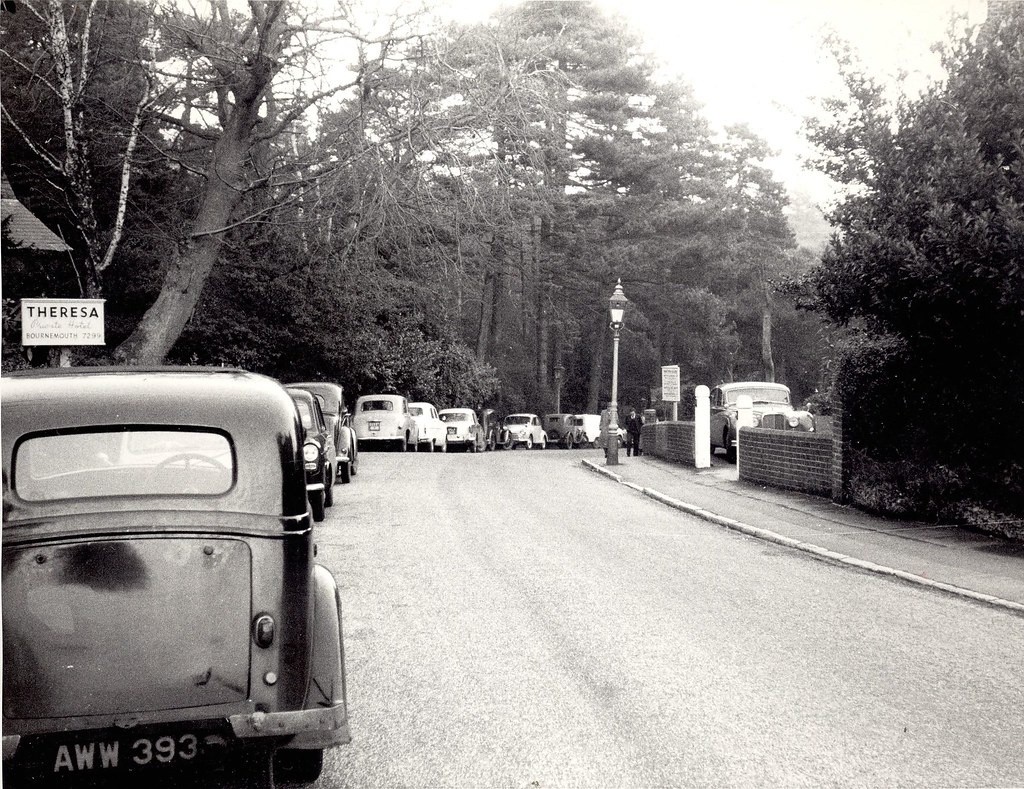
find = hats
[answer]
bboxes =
[607,402,611,407]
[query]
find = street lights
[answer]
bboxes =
[604,276,627,466]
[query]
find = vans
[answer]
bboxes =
[575,414,625,449]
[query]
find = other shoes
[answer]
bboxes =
[605,454,608,458]
[634,454,638,456]
[627,452,630,456]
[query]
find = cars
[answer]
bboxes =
[503,413,548,450]
[289,388,338,524]
[282,381,359,483]
[542,413,588,448]
[474,408,512,452]
[352,394,421,453]
[2,362,352,789]
[436,408,488,453]
[405,402,449,452]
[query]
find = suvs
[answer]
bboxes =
[709,381,814,462]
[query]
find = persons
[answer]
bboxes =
[599,402,611,458]
[625,407,642,457]
